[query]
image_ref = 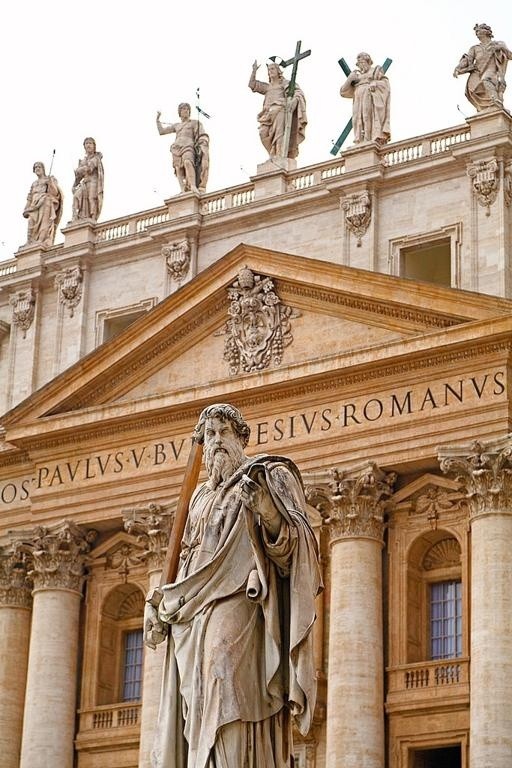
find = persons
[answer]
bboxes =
[141,404,326,767]
[339,53,394,146]
[453,23,512,112]
[68,136,104,223]
[23,162,62,247]
[248,56,306,160]
[154,100,210,195]
